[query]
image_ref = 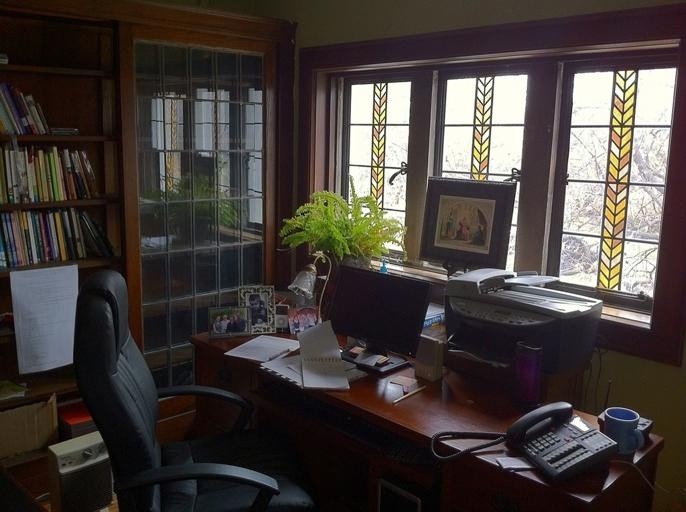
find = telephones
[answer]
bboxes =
[504,402,617,481]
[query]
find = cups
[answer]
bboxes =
[604,406,644,463]
[512,342,542,403]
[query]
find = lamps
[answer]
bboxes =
[288,254,332,324]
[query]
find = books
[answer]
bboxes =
[0,77,115,265]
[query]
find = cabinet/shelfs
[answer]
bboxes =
[0,0,299,445]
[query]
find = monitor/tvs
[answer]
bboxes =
[324,265,433,376]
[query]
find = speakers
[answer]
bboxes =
[413,334,445,383]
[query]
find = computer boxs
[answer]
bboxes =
[377,472,441,512]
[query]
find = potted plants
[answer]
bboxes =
[141,172,241,242]
[278,173,409,270]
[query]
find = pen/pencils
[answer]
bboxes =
[392,385,426,404]
[269,349,290,360]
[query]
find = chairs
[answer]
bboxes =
[74,270,314,512]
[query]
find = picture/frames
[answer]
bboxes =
[418,177,517,269]
[237,286,276,334]
[207,307,251,338]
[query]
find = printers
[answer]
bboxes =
[444,268,604,376]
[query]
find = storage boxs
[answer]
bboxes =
[0,395,59,468]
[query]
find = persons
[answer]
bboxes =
[213,312,246,333]
[444,212,483,244]
[249,294,267,326]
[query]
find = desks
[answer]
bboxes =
[189,330,664,512]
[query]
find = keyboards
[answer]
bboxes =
[266,383,385,452]
